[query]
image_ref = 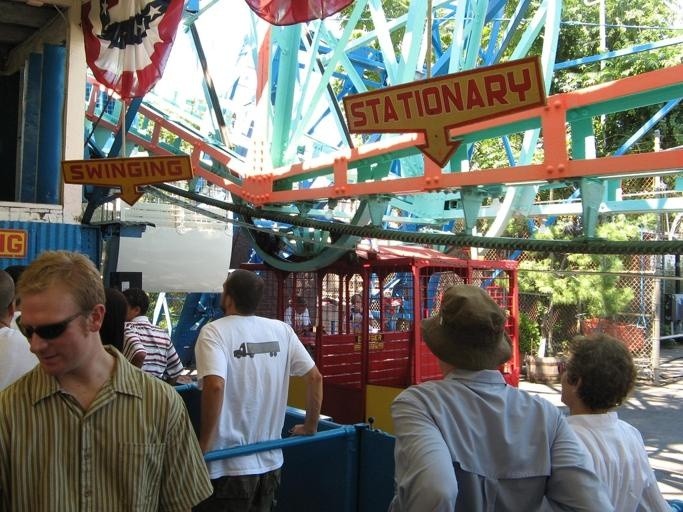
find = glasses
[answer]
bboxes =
[558,361,569,373]
[16,313,82,339]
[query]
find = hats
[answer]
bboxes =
[419,285,513,371]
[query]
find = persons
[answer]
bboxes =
[283,292,381,335]
[387,282,618,512]
[1,249,219,512]
[193,268,326,512]
[537,331,676,511]
[0,258,186,388]
